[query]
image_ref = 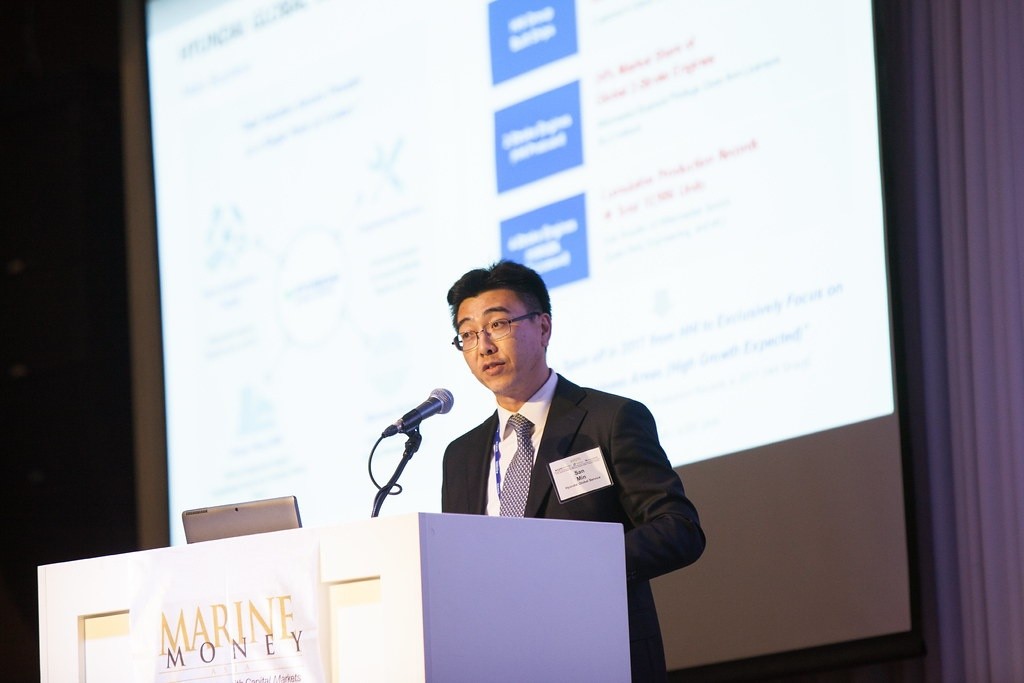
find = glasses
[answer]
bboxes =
[452,312,541,352]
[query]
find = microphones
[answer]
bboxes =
[382,388,454,439]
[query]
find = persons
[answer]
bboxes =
[439,258,708,683]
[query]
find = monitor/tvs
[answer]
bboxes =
[181,496,302,544]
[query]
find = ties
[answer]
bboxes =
[499,414,535,518]
